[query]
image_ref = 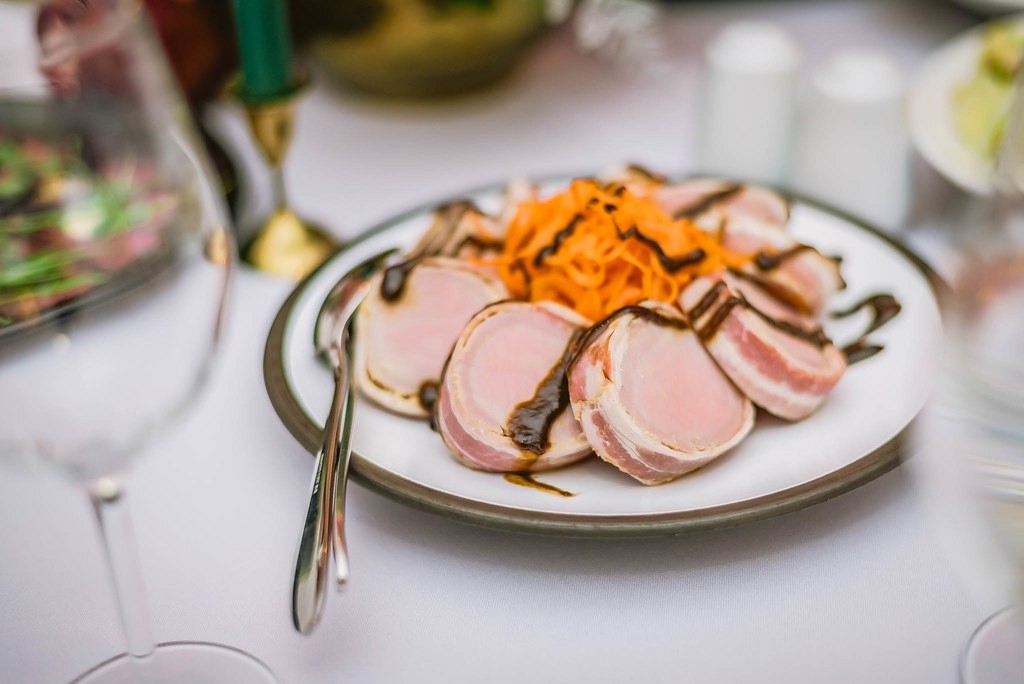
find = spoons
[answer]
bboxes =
[290,247,401,637]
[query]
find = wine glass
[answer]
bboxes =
[934,184,1024,684]
[0,0,283,684]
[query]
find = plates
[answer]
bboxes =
[263,170,957,537]
[907,13,1024,203]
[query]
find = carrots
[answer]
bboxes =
[444,178,776,329]
